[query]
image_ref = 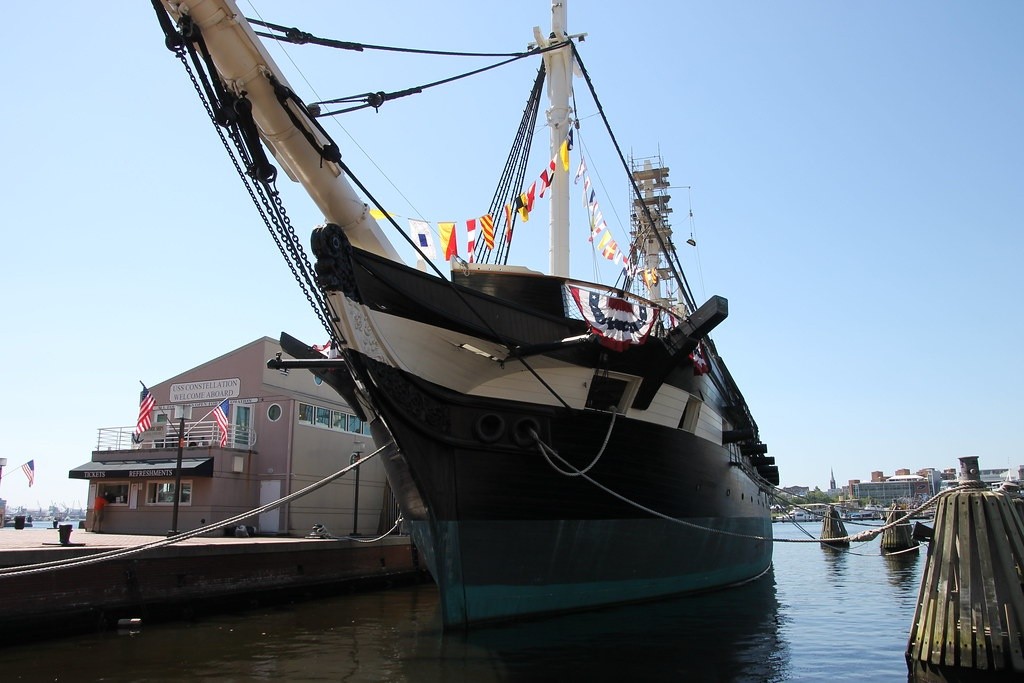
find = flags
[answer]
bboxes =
[368,127,576,264]
[135,386,157,435]
[574,155,660,292]
[22,459,34,487]
[212,399,229,448]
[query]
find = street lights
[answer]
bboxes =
[166,404,192,539]
[349,439,365,537]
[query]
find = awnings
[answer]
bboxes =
[68,456,215,479]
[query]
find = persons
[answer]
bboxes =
[91,491,109,534]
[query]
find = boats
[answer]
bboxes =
[152,0,780,632]
